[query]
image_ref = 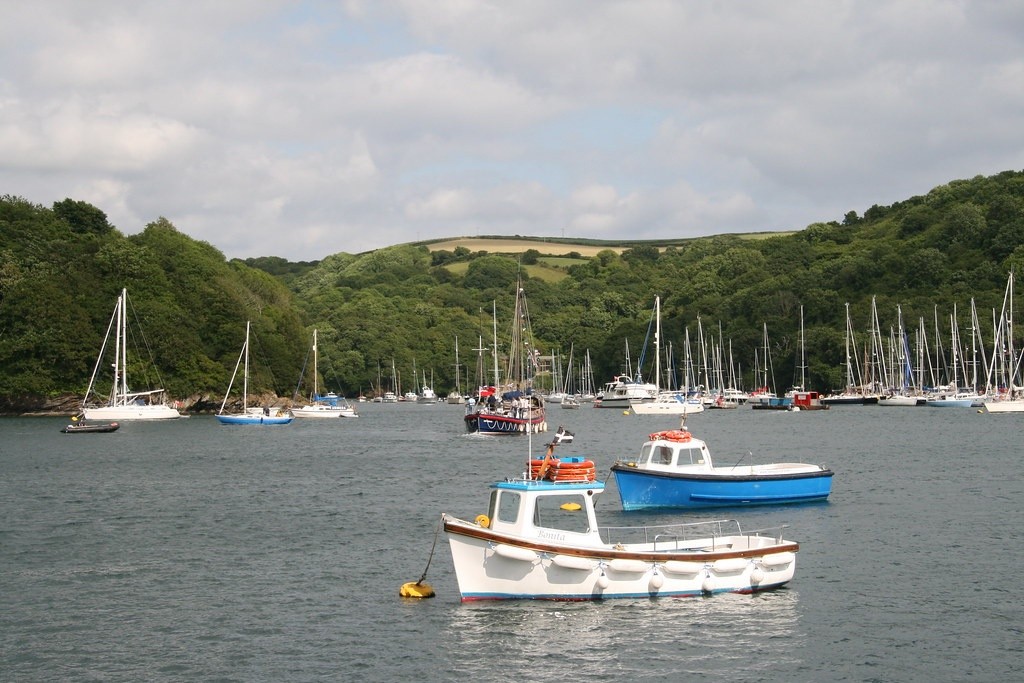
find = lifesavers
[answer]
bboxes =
[648,429,667,439]
[526,459,559,480]
[551,459,595,482]
[666,430,692,443]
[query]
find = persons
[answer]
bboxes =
[488,395,496,411]
[511,397,518,418]
[518,396,525,419]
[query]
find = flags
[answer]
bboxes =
[552,427,576,444]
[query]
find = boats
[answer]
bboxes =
[607,403,835,512]
[440,423,801,606]
[59,421,120,433]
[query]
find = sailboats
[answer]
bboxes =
[214,321,295,426]
[78,288,182,422]
[287,331,357,419]
[356,271,1024,416]
[462,253,546,434]
